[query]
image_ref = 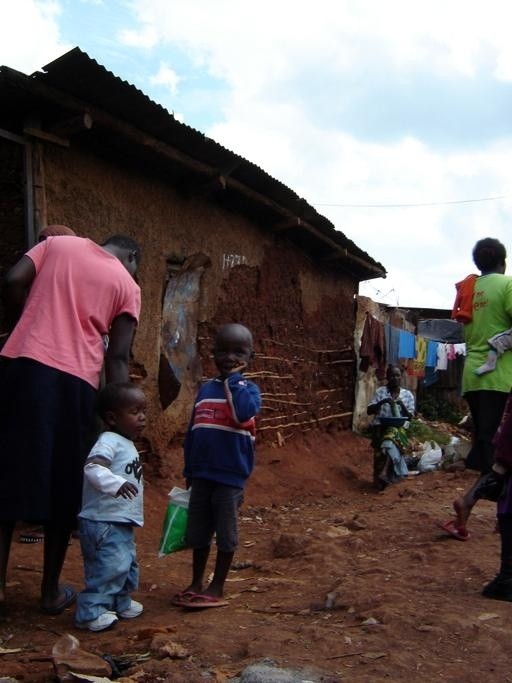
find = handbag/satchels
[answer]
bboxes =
[159,486,192,557]
[417,440,442,472]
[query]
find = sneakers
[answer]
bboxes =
[75,600,144,632]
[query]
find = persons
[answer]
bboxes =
[434,239,512,540]
[484,392,512,601]
[473,327,512,375]
[74,381,147,633]
[170,323,261,609]
[19,224,76,544]
[0,234,142,614]
[367,366,415,490]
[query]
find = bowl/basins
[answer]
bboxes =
[377,417,409,427]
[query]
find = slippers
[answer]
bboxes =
[47,586,75,611]
[437,519,470,540]
[172,592,228,608]
